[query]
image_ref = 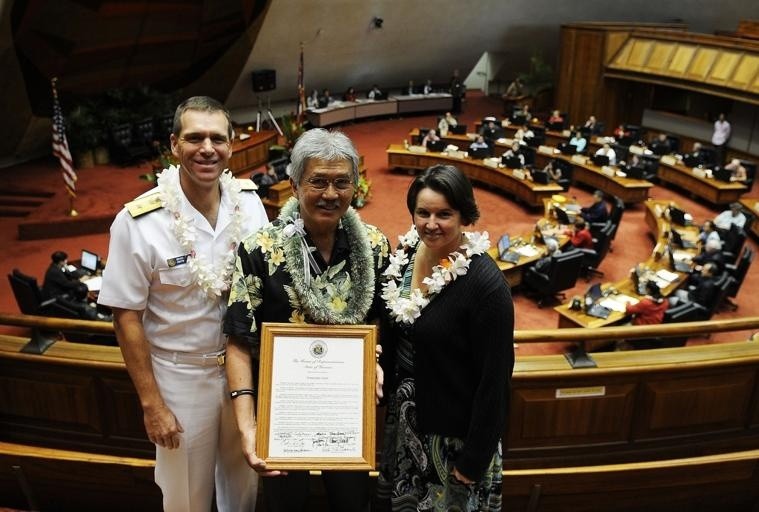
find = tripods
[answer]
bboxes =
[265,94,285,137]
[249,94,274,133]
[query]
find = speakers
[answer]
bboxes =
[254,69,276,92]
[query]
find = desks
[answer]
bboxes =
[307,92,453,129]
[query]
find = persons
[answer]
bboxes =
[371,162,516,511]
[367,84,382,100]
[221,125,393,512]
[39,249,79,294]
[306,88,320,109]
[535,188,604,275]
[95,92,274,512]
[504,74,525,97]
[91,297,113,322]
[428,108,747,184]
[66,281,95,321]
[623,203,745,326]
[319,89,333,109]
[342,87,357,102]
[407,80,465,114]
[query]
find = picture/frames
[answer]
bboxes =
[254,322,376,475]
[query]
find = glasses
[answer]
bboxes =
[302,176,356,189]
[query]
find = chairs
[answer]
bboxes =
[228,123,293,217]
[8,251,113,323]
[379,110,758,347]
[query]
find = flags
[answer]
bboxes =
[48,82,80,200]
[296,50,307,128]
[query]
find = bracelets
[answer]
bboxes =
[229,388,255,399]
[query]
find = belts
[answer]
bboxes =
[149,343,226,368]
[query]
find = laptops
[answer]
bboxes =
[78,249,100,277]
[318,88,733,320]
